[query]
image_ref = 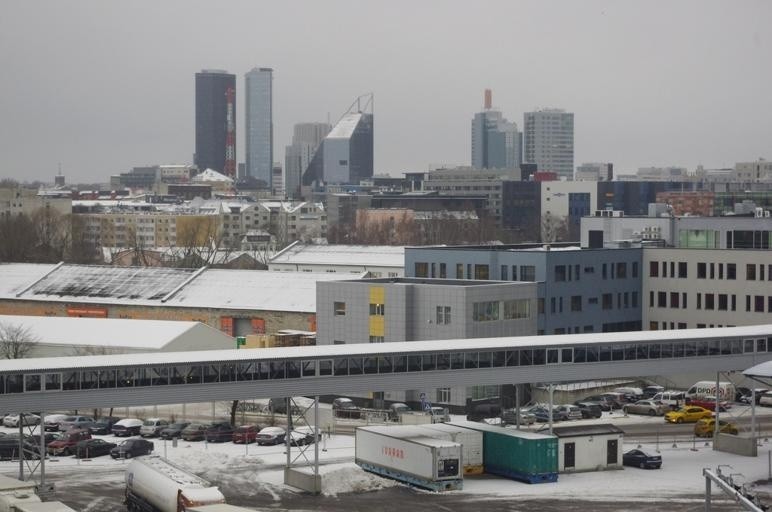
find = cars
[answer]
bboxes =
[3,412,41,428]
[160,421,191,440]
[694,419,738,439]
[181,423,208,442]
[47,428,93,456]
[503,381,772,426]
[233,425,260,444]
[426,406,453,423]
[89,416,121,435]
[111,418,145,437]
[622,445,663,470]
[43,413,68,431]
[284,425,322,447]
[259,405,301,416]
[58,416,96,433]
[230,401,262,414]
[75,438,117,459]
[0,431,61,459]
[388,402,412,423]
[140,418,169,438]
[111,438,154,460]
[254,427,287,446]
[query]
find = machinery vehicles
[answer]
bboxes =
[123,452,226,512]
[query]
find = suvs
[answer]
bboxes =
[207,422,236,443]
[332,398,360,419]
